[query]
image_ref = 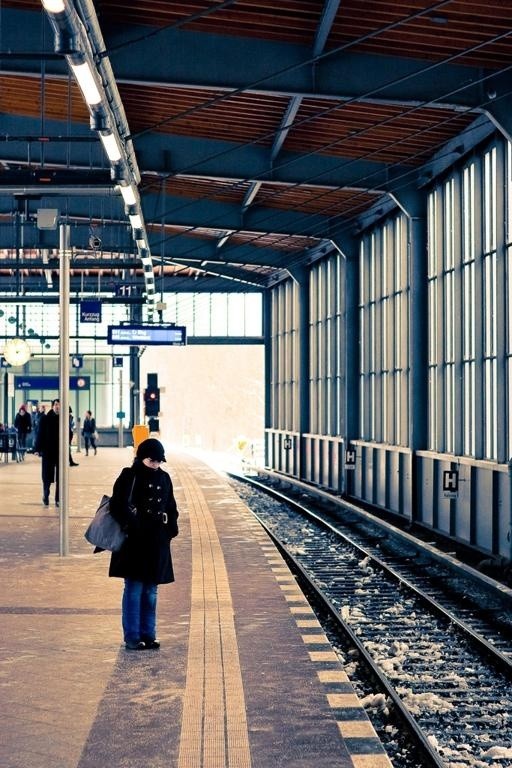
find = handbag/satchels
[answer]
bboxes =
[84,494,138,553]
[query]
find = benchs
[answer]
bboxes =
[0,433,26,463]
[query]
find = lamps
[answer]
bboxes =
[41,0,157,324]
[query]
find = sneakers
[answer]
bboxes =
[126,639,160,650]
[44,498,60,507]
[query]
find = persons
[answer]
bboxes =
[81,409,99,457]
[92,437,179,651]
[68,405,79,467]
[33,397,60,506]
[12,402,32,456]
[29,403,47,456]
[0,422,11,453]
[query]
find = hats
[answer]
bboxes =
[138,438,166,463]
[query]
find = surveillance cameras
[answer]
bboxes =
[89,235,102,251]
